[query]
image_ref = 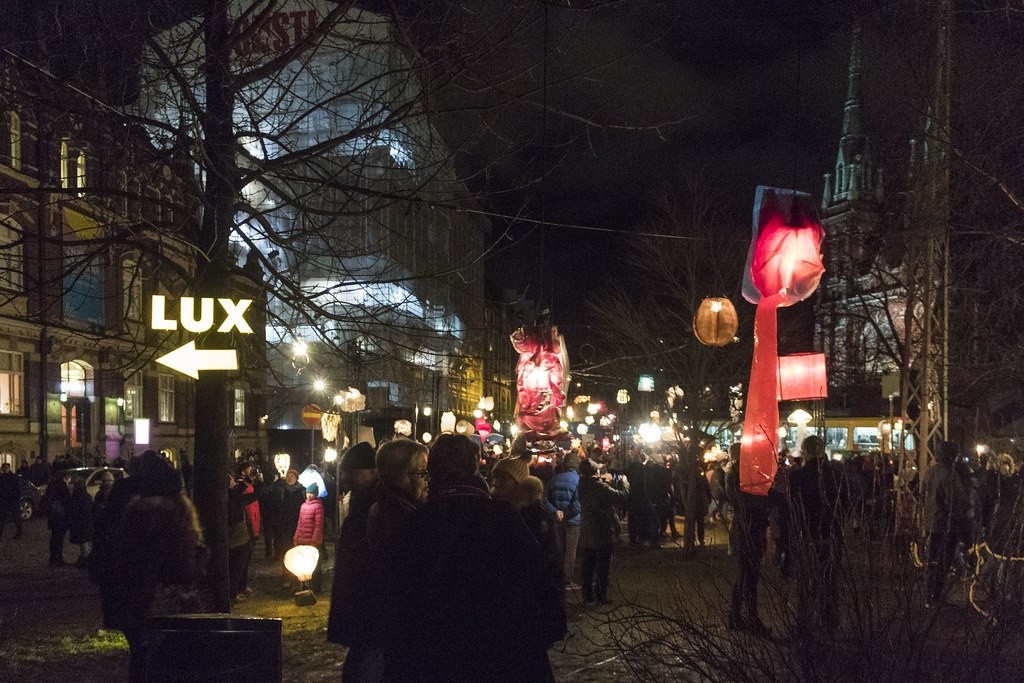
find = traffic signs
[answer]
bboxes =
[147,292,269,381]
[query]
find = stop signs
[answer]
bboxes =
[300,404,323,427]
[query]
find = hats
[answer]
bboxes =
[492,451,532,485]
[129,451,169,493]
[343,442,378,469]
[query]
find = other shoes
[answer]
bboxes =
[50,559,66,567]
[243,587,253,592]
[729,616,770,637]
[13,531,22,540]
[234,596,244,603]
[598,599,612,605]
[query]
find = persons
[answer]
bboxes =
[0,439,351,683]
[327,441,384,682]
[490,451,568,683]
[478,436,731,604]
[364,439,431,546]
[724,442,775,633]
[841,441,1024,609]
[773,436,852,630]
[374,433,556,683]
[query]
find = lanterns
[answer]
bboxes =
[278,188,828,580]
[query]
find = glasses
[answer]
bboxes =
[410,468,430,478]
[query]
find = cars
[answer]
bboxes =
[15,466,129,520]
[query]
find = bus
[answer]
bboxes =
[696,416,917,471]
